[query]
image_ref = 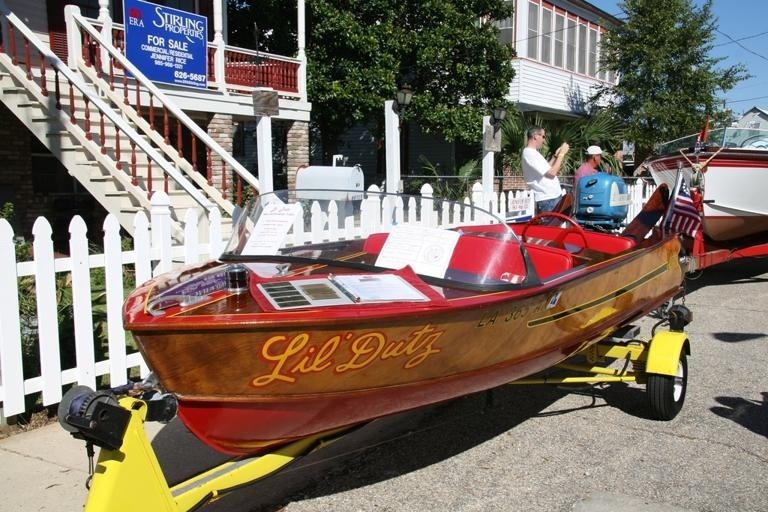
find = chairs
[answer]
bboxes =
[357,220,637,304]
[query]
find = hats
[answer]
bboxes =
[584,145,604,155]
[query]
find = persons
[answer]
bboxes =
[521,124,571,216]
[573,144,604,202]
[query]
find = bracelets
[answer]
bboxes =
[554,155,557,159]
[559,152,565,158]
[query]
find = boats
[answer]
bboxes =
[642,128,768,241]
[122,189,682,462]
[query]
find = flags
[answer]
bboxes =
[658,174,702,240]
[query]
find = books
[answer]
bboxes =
[256,269,434,310]
